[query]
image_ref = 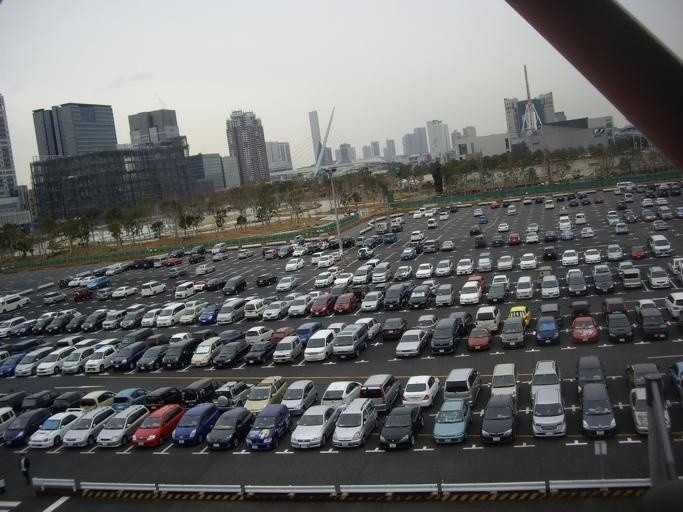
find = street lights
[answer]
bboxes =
[325,168,345,260]
